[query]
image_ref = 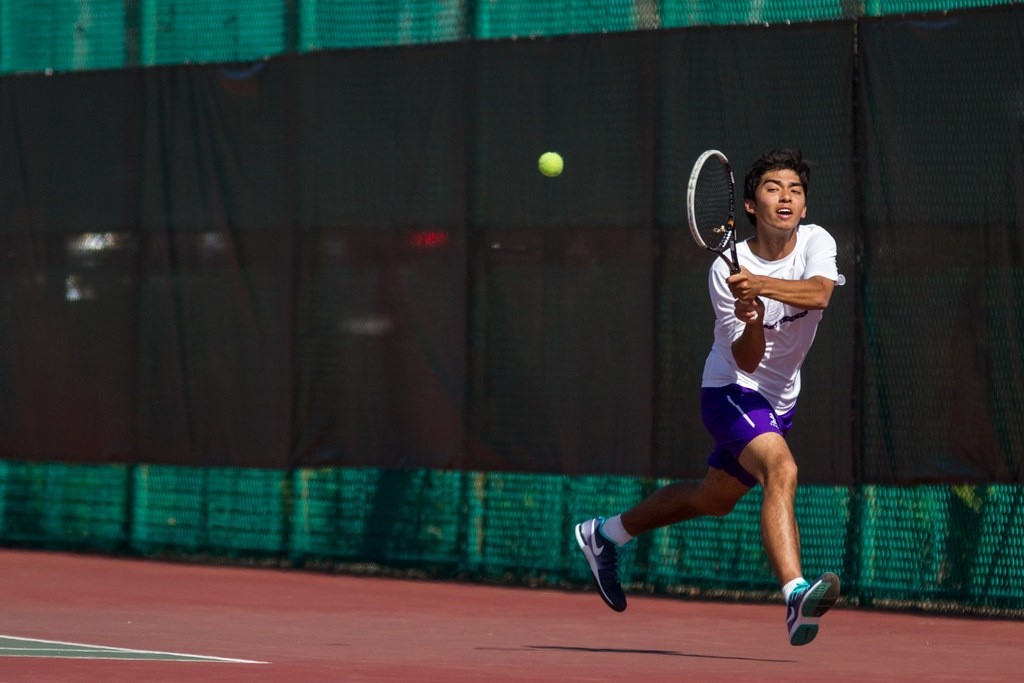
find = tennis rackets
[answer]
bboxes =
[685,148,742,276]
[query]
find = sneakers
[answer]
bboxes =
[786,572,840,645]
[574,517,627,612]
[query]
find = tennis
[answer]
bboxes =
[538,152,564,178]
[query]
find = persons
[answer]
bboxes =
[574,148,839,646]
